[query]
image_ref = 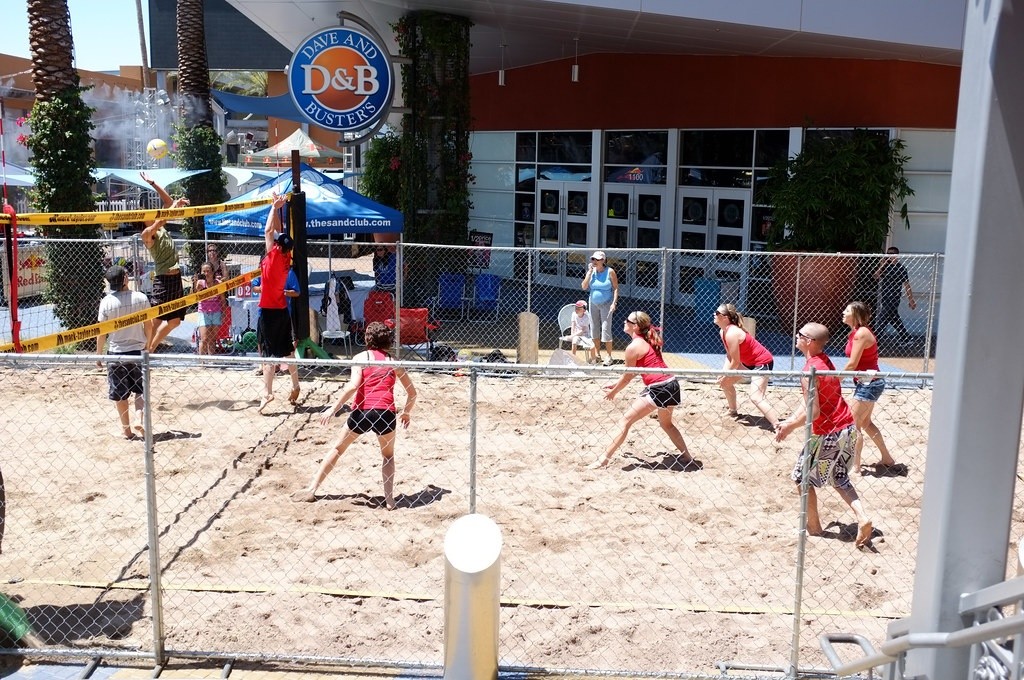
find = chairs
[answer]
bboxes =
[431,274,467,322]
[467,274,503,324]
[195,297,248,356]
[359,291,396,347]
[384,308,438,361]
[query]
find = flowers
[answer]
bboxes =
[388,156,401,170]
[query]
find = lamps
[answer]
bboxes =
[498,45,506,86]
[572,38,579,82]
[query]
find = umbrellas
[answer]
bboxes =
[251,127,346,158]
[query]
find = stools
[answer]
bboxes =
[320,330,354,360]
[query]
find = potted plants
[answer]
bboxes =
[355,123,427,256]
[757,125,916,336]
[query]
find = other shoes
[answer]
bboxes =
[604,356,612,366]
[588,354,601,364]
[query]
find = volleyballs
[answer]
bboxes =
[147,138,168,160]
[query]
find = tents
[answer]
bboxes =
[201,161,404,310]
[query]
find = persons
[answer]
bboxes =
[255,192,300,411]
[775,322,873,547]
[571,300,598,363]
[293,321,416,509]
[129,171,189,353]
[206,243,229,306]
[193,261,226,356]
[836,301,894,476]
[373,241,396,295]
[713,303,781,435]
[585,310,692,469]
[874,246,915,345]
[96,264,152,438]
[581,251,619,365]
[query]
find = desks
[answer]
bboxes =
[161,284,372,345]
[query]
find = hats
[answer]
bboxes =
[571,300,588,309]
[106,265,125,289]
[274,233,294,249]
[590,251,606,260]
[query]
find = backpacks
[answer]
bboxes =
[480,349,518,379]
[427,346,457,374]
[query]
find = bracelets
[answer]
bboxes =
[613,302,617,305]
[150,181,154,185]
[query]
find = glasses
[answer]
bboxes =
[592,257,604,261]
[715,310,726,317]
[376,248,385,251]
[207,249,219,253]
[626,317,639,326]
[798,330,816,341]
